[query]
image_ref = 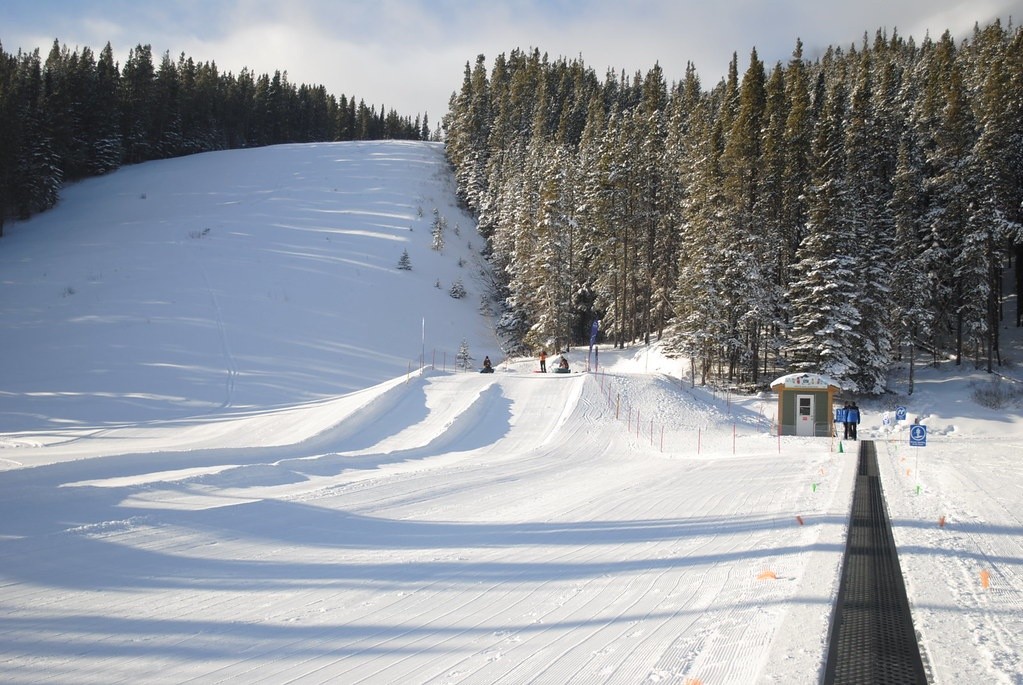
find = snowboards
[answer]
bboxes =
[533,371,547,373]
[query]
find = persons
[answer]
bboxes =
[484,356,491,367]
[539,348,547,373]
[842,401,860,441]
[559,356,569,369]
